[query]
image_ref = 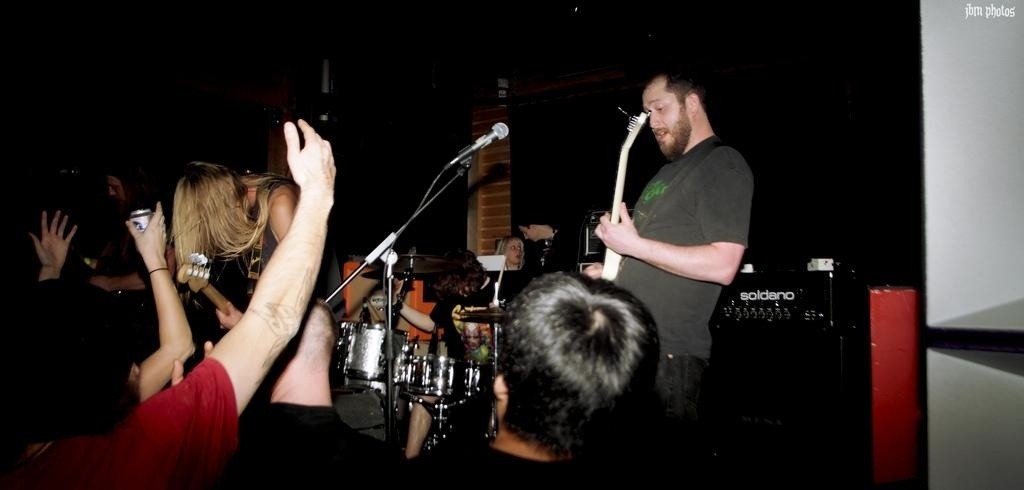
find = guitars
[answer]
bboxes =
[599,106,652,280]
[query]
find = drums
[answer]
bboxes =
[331,382,388,442]
[333,320,411,419]
[409,355,466,398]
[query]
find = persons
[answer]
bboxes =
[408,271,681,490]
[404,216,580,458]
[4,119,337,486]
[580,64,752,457]
[2,160,300,468]
[210,297,406,488]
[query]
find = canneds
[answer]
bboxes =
[129,208,152,231]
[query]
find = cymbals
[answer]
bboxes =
[456,307,508,320]
[358,252,449,274]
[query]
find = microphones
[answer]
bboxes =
[440,122,509,171]
